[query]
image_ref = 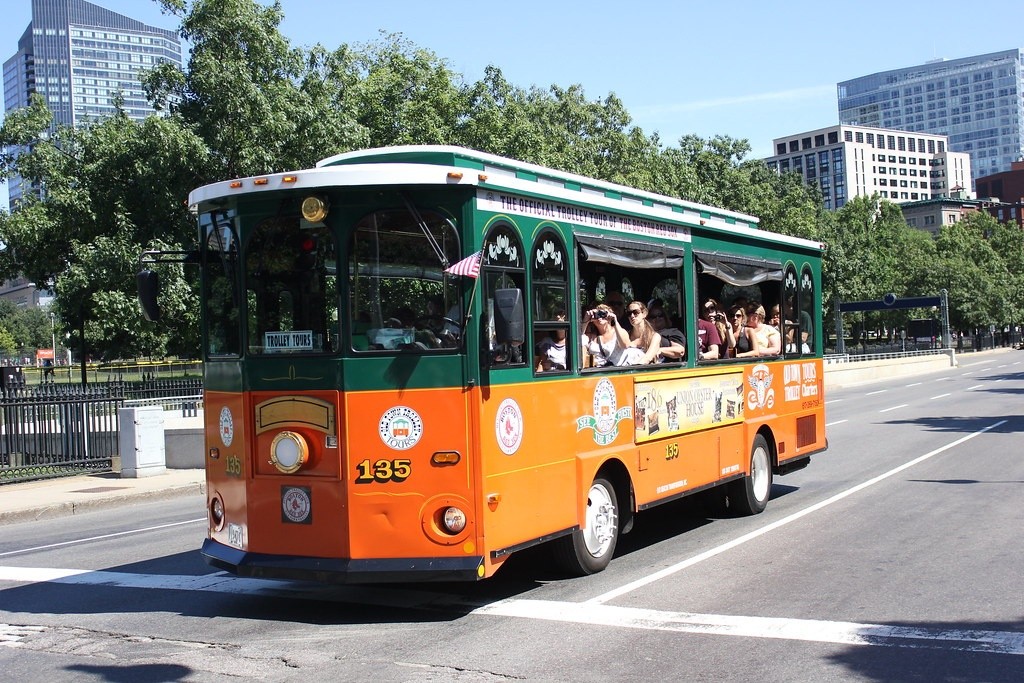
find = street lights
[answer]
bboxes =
[932,306,938,355]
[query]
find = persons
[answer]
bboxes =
[44,360,55,380]
[536,291,686,372]
[698,297,813,360]
[440,272,526,347]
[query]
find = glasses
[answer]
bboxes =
[730,314,741,318]
[771,312,779,317]
[703,304,715,315]
[627,309,640,317]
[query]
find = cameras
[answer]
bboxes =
[594,309,607,319]
[709,315,723,321]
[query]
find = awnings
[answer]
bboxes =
[37,350,54,359]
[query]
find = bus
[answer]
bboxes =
[134,144,829,585]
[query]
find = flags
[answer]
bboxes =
[443,250,482,279]
